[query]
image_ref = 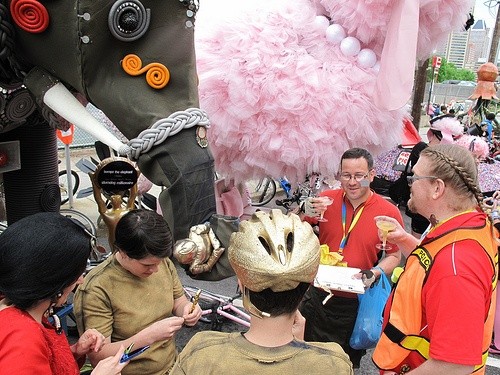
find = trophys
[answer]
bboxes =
[88,156,141,254]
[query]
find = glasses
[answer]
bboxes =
[406,175,439,187]
[340,168,372,182]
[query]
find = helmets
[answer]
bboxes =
[228,207,321,292]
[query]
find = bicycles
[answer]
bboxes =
[243,172,319,213]
[56,159,79,205]
[57,207,112,279]
[182,284,252,329]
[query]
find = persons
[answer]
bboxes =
[294,147,404,369]
[170,209,353,375]
[81,209,202,375]
[424,102,500,166]
[372,145,500,375]
[0,211,130,375]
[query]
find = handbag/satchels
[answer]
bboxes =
[350,266,392,349]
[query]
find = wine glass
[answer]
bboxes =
[318,197,333,221]
[376,216,396,250]
